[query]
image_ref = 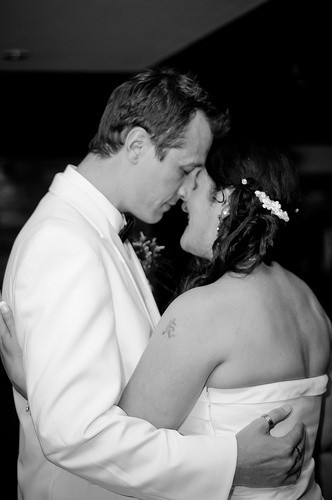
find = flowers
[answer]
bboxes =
[131,231,164,272]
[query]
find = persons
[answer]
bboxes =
[0,129,332,500]
[0,71,308,500]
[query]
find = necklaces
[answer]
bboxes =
[248,256,261,261]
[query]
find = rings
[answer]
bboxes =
[294,446,302,461]
[262,413,274,430]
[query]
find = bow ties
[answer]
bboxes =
[119,219,137,244]
